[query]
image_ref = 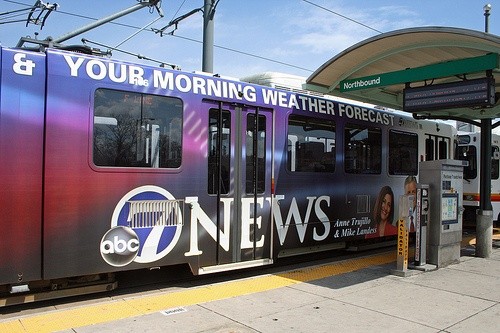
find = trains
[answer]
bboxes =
[0,38,499,308]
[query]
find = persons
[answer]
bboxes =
[364,185,399,239]
[395,175,419,234]
